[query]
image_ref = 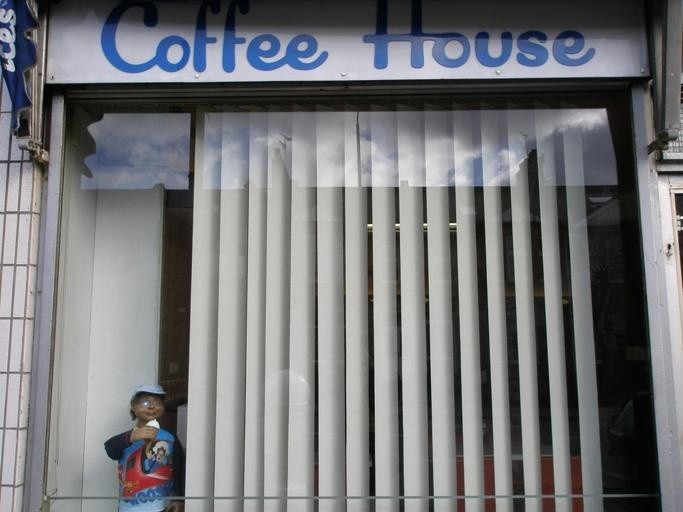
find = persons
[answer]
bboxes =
[104,384,185,512]
